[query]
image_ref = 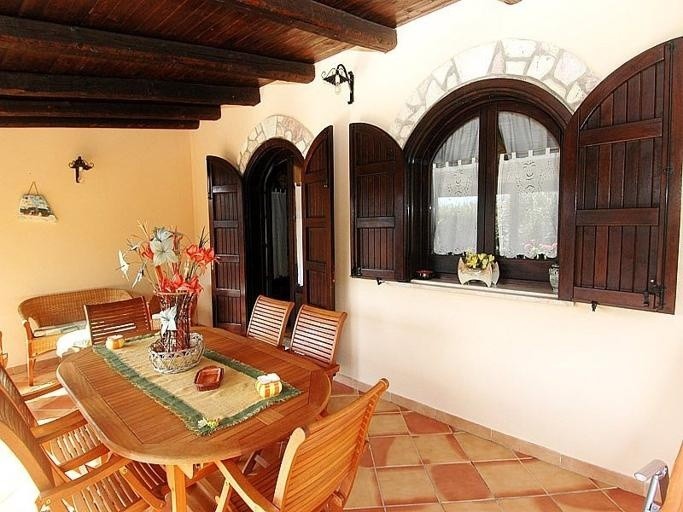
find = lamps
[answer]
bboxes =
[67,153,95,184]
[320,62,355,106]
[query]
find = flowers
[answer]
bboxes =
[114,216,220,293]
[462,251,494,270]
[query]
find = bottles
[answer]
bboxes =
[549,265,558,293]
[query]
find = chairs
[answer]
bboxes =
[215,378,389,511]
[0,294,348,512]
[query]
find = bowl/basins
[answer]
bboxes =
[416,270,433,279]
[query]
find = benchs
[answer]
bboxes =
[18,287,134,386]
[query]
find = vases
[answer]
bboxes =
[457,257,500,287]
[547,263,559,294]
